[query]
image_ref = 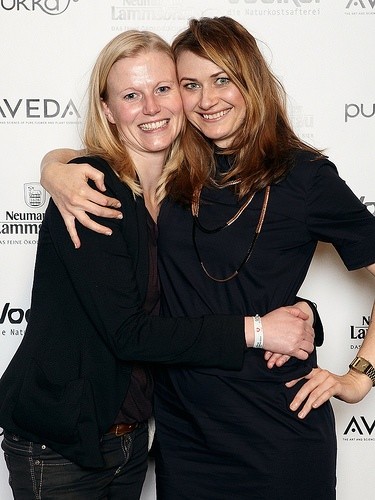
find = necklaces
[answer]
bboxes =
[188,144,272,281]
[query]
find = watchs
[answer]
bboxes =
[348,356,375,389]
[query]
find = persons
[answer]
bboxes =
[36,14,375,500]
[0,27,326,500]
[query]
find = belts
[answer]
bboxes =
[102,423,146,436]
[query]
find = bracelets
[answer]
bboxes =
[250,314,265,350]
